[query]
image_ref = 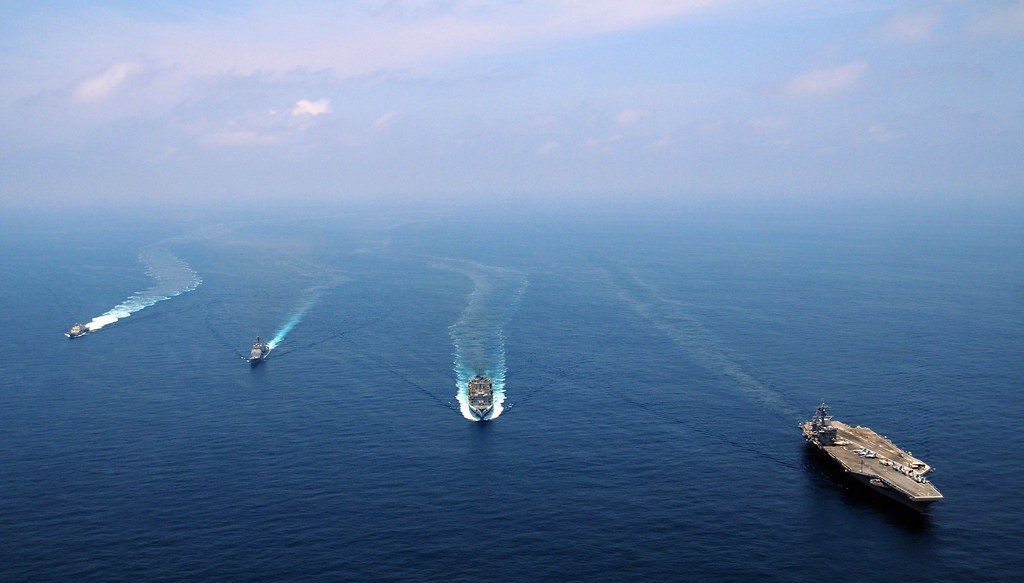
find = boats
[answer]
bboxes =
[467,374,493,421]
[798,400,944,514]
[69,322,89,337]
[250,336,270,362]
